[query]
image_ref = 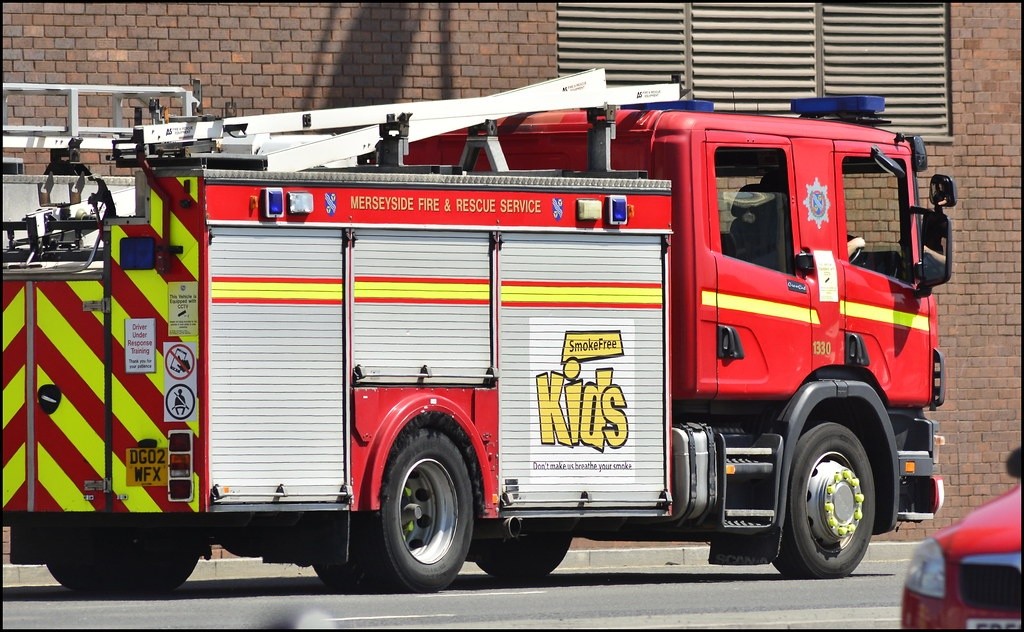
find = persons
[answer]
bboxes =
[760,171,865,260]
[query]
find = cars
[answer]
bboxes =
[899,448,1024,632]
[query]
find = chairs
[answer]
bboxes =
[728,185,794,274]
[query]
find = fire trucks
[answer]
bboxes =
[1,68,959,599]
